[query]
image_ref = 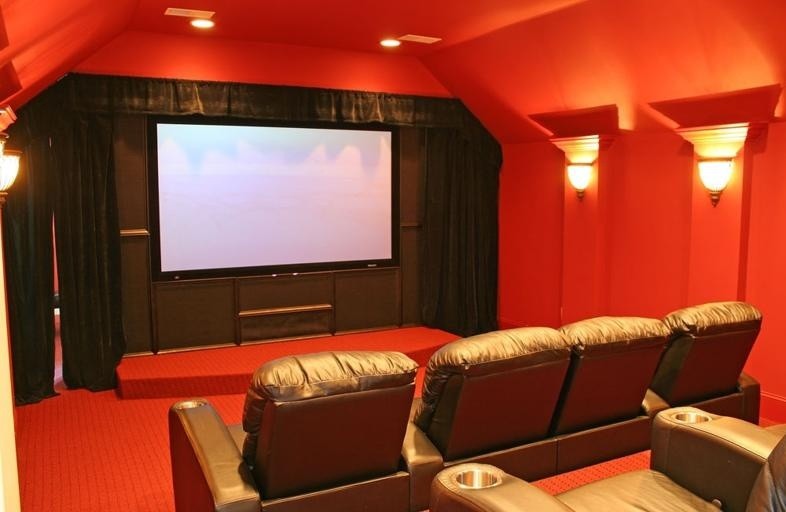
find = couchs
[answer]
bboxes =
[168,299,786,512]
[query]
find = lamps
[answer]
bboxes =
[567,159,595,201]
[0,129,22,210]
[695,157,732,208]
